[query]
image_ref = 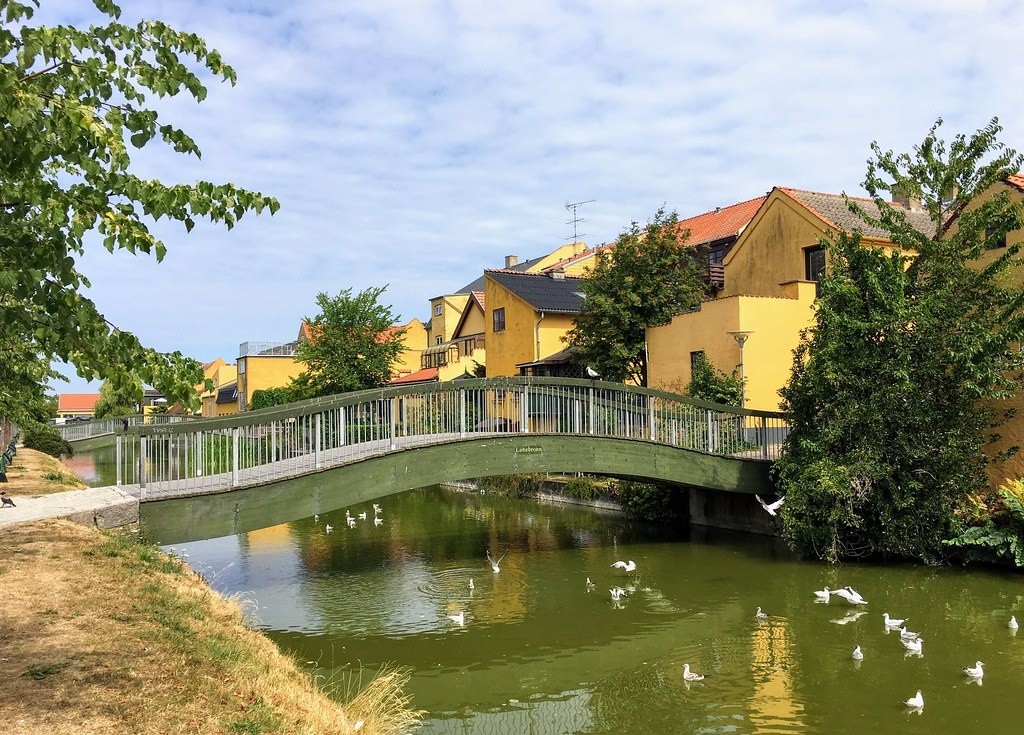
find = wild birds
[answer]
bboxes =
[851,644,863,659]
[813,586,869,605]
[372,503,383,522]
[585,577,596,587]
[882,613,924,650]
[756,607,769,618]
[902,689,924,707]
[346,509,366,529]
[609,560,637,572]
[448,611,465,622]
[681,664,710,681]
[467,579,475,589]
[326,524,334,530]
[608,587,627,601]
[960,661,985,677]
[755,494,785,517]
[586,367,600,382]
[487,549,508,574]
[315,514,319,519]
[1007,615,1018,629]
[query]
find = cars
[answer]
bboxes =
[65,415,93,425]
